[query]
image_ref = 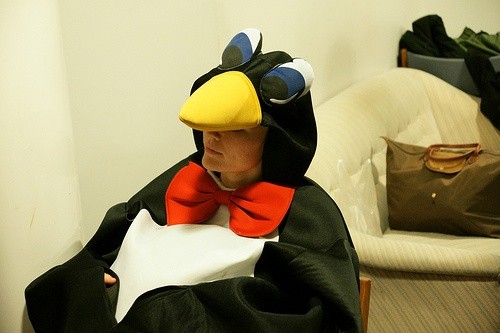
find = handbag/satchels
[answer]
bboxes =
[379,136,500,238]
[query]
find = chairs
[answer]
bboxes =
[401,48,500,99]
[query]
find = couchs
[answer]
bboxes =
[304,66,500,332]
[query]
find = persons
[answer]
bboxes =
[23,27,366,332]
